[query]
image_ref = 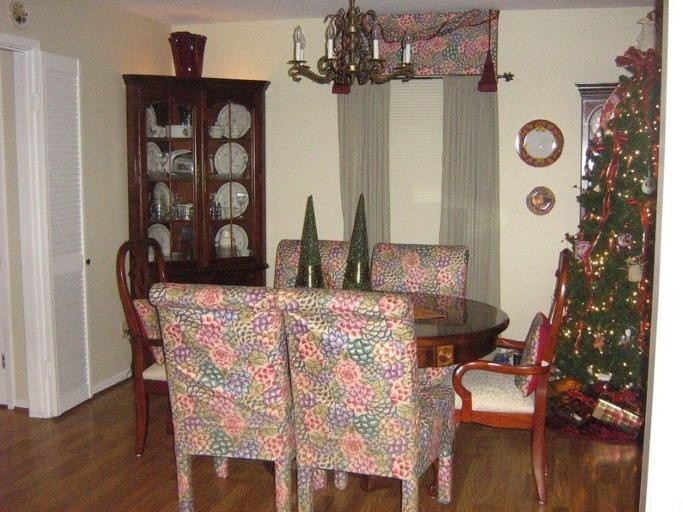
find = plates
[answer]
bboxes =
[207,101,254,257]
[145,101,195,262]
[525,187,557,215]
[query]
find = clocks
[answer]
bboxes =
[10,0,30,28]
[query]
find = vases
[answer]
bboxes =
[168,31,207,77]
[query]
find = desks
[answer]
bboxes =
[263,290,510,492]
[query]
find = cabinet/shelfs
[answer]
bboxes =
[574,81,624,230]
[121,73,271,380]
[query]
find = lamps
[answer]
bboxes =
[286,0,415,94]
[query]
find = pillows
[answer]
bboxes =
[513,313,551,398]
[133,297,165,365]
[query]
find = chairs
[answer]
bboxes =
[274,286,458,512]
[273,239,349,291]
[148,283,327,512]
[118,234,170,458]
[427,249,571,504]
[370,241,469,390]
